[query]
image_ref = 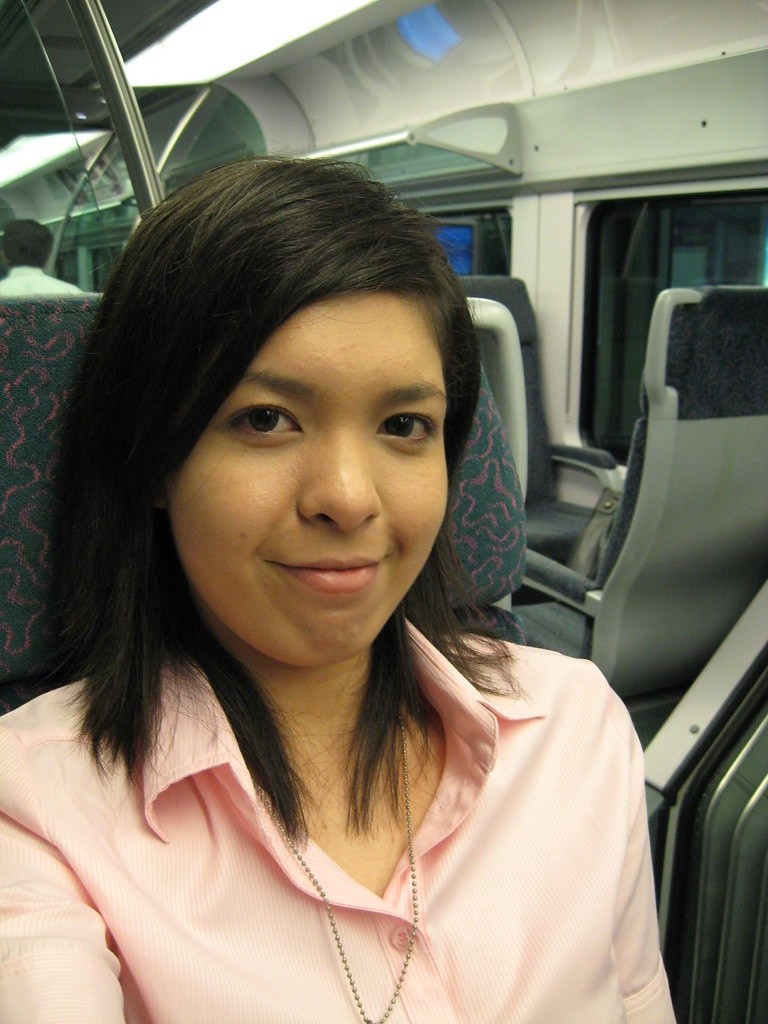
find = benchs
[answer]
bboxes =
[1,272,768,1024]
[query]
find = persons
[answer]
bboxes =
[0,219,83,296]
[0,156,677,1024]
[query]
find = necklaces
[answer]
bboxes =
[250,709,418,1024]
